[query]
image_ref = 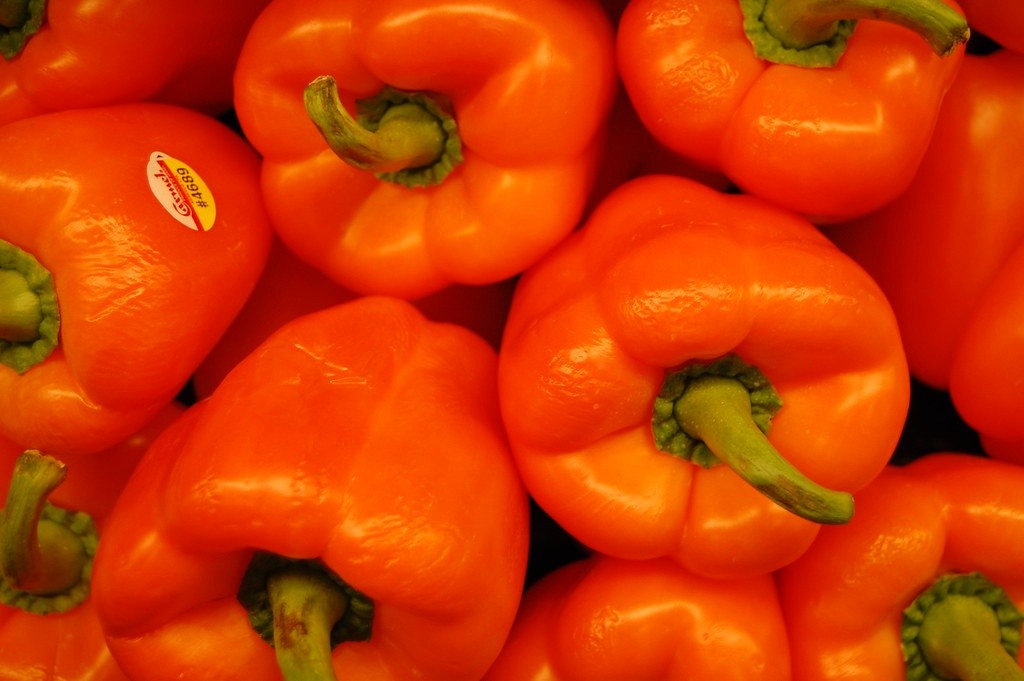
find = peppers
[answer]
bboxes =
[0,0,1024,681]
